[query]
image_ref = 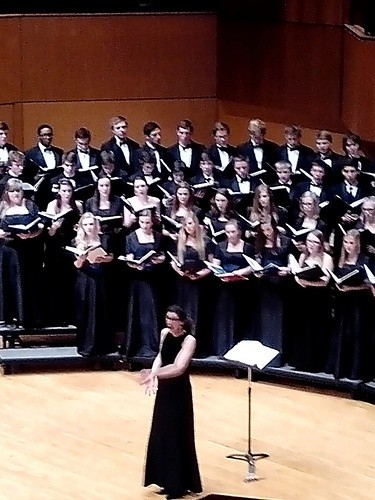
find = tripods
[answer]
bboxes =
[218,348,280,467]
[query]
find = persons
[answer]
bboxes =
[41,178,82,326]
[252,217,299,366]
[69,212,115,358]
[357,198,375,277]
[167,212,215,353]
[120,210,168,357]
[0,114,375,265]
[212,220,256,359]
[139,304,202,500]
[0,178,43,328]
[291,231,334,373]
[327,230,366,382]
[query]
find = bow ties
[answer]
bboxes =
[287,145,299,151]
[204,178,211,182]
[217,146,227,151]
[237,174,250,183]
[352,156,361,161]
[253,145,262,148]
[44,148,52,152]
[152,148,157,151]
[80,150,88,154]
[120,140,127,146]
[280,180,289,186]
[181,145,191,150]
[323,156,331,160]
[310,183,320,187]
[144,174,151,176]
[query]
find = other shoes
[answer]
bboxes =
[165,489,186,500]
[155,488,170,495]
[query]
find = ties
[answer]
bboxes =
[349,187,353,195]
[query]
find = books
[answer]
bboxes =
[285,222,312,243]
[8,217,42,235]
[118,250,157,266]
[37,209,76,222]
[121,194,158,216]
[167,251,364,289]
[65,244,109,263]
[224,338,281,369]
[92,213,123,225]
